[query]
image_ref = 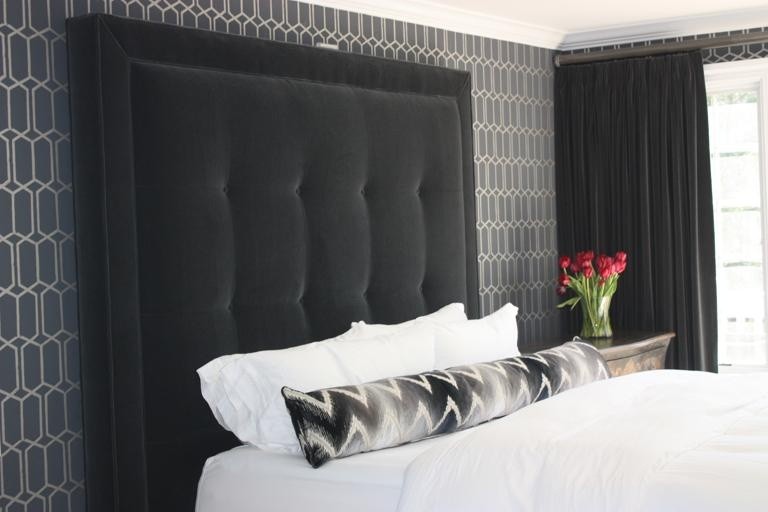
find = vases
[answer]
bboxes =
[578,296,613,337]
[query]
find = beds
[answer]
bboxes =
[64,12,768,512]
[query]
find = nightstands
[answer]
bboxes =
[520,328,676,375]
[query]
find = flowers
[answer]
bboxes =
[555,250,628,338]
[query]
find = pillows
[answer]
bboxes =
[350,304,471,341]
[280,334,608,470]
[195,321,411,459]
[389,304,520,376]
